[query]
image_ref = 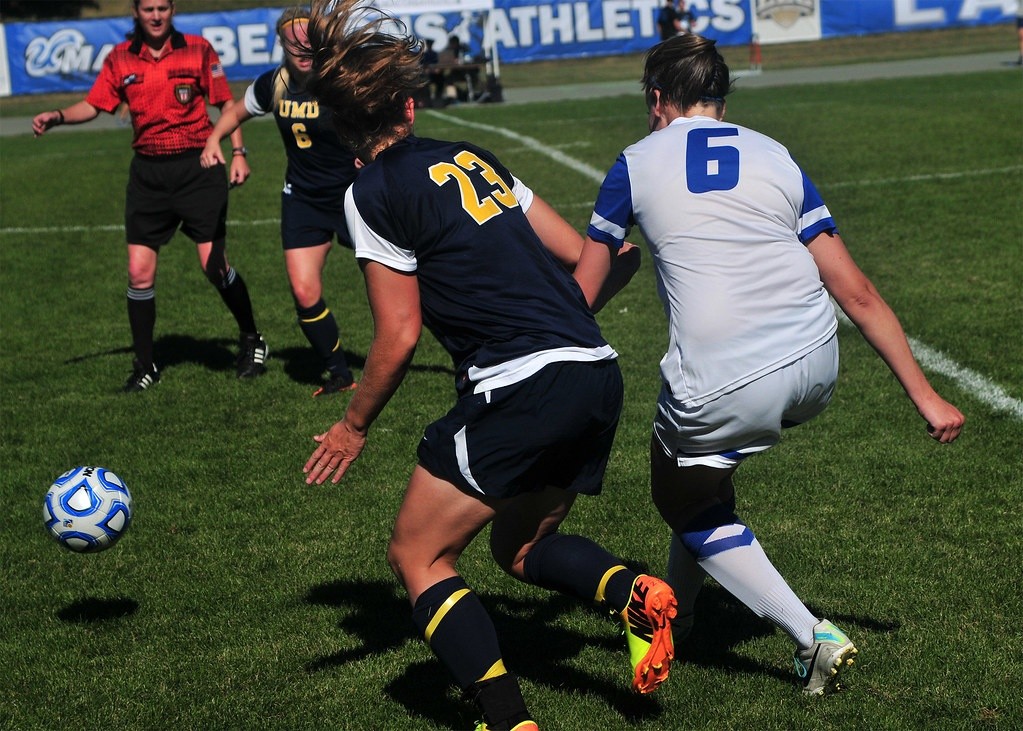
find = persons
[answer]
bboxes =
[657,0,678,40]
[417,39,444,101]
[674,0,696,37]
[278,0,678,731]
[573,35,965,698]
[33,0,271,395]
[201,10,357,399]
[438,36,478,102]
[1016,16,1023,66]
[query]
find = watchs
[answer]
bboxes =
[232,146,247,156]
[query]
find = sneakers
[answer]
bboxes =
[312,371,357,399]
[235,333,271,381]
[795,619,858,699]
[125,358,160,393]
[619,574,676,696]
[473,722,540,731]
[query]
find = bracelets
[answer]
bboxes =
[233,154,246,157]
[56,108,64,125]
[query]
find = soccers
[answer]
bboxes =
[43,466,134,552]
